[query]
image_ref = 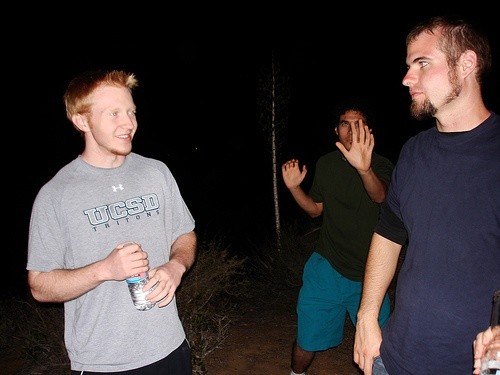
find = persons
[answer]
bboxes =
[352,16,500,374]
[24,68,208,374]
[280,99,409,375]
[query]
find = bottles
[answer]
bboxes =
[124,244,154,311]
[482,290,500,375]
